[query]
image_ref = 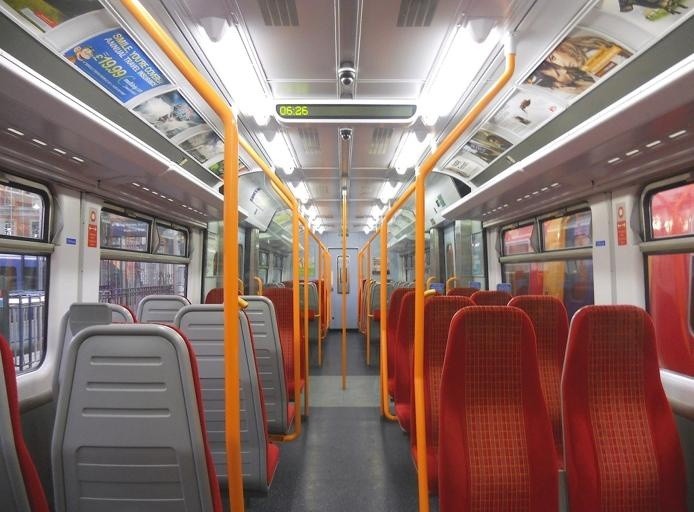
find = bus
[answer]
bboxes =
[0,254,122,355]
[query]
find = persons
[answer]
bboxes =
[531,62,594,94]
[150,111,176,129]
[543,36,632,82]
[486,135,512,152]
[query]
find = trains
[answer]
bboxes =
[408,183,693,418]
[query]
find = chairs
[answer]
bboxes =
[136,292,191,331]
[231,293,298,438]
[354,280,516,434]
[45,319,225,512]
[502,292,571,446]
[170,304,284,492]
[203,281,336,401]
[407,294,483,487]
[49,300,142,445]
[565,303,682,512]
[0,336,50,510]
[435,303,562,512]
